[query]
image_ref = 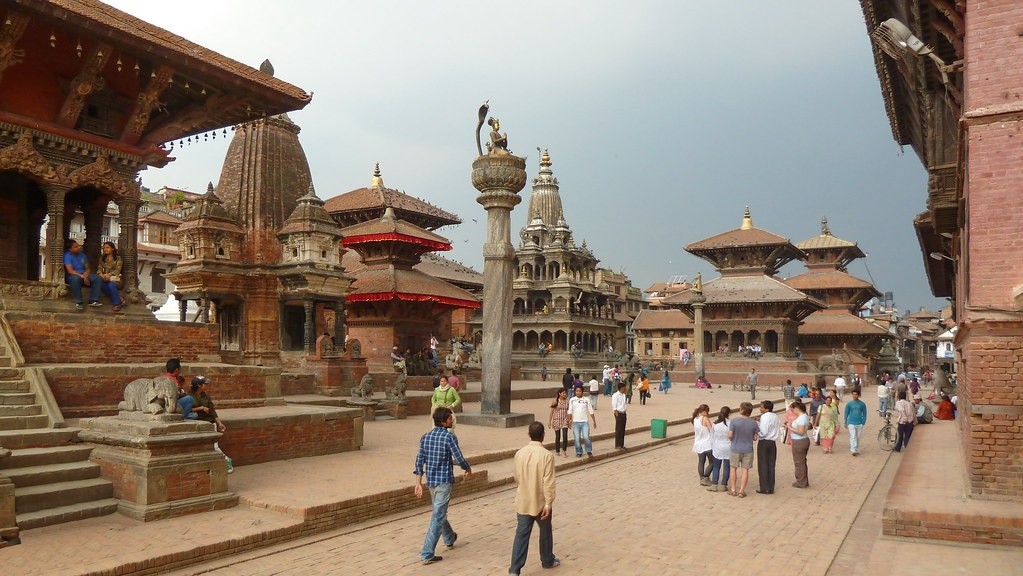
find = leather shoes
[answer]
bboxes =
[770,488,773,494]
[756,488,770,494]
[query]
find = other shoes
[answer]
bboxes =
[616,446,628,451]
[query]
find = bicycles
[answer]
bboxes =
[877,409,899,451]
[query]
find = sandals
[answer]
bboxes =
[739,491,747,498]
[727,489,738,496]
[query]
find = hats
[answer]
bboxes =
[191,375,213,387]
[912,394,922,400]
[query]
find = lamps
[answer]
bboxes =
[870,15,966,83]
[930,251,956,273]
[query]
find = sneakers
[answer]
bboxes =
[555,451,560,456]
[700,476,704,485]
[587,452,593,459]
[562,451,568,457]
[118,296,127,306]
[792,482,801,488]
[113,303,121,310]
[89,300,103,307]
[719,484,728,492]
[447,531,458,549]
[548,558,560,567]
[424,555,443,565]
[576,453,582,457]
[794,481,809,486]
[706,484,717,492]
[703,477,712,485]
[74,304,84,310]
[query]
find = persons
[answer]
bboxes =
[96,242,126,312]
[413,407,472,564]
[691,404,715,486]
[756,400,780,495]
[177,375,186,395]
[63,240,103,310]
[187,375,234,474]
[487,118,511,154]
[705,406,733,491]
[728,402,761,498]
[390,304,958,459]
[507,421,560,576]
[782,401,810,489]
[162,358,194,419]
